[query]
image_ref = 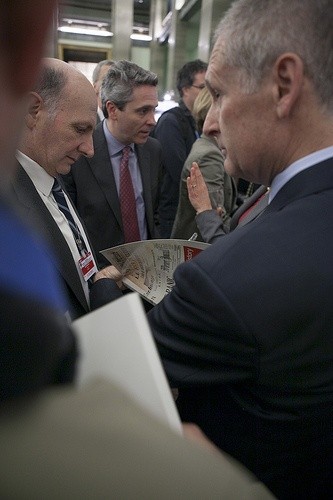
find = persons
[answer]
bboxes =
[0,0,333,500]
[11,52,100,336]
[59,59,169,314]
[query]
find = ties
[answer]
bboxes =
[52,176,95,290]
[119,147,141,243]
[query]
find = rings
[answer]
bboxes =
[192,185,197,188]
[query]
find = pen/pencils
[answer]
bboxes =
[189,232,198,241]
